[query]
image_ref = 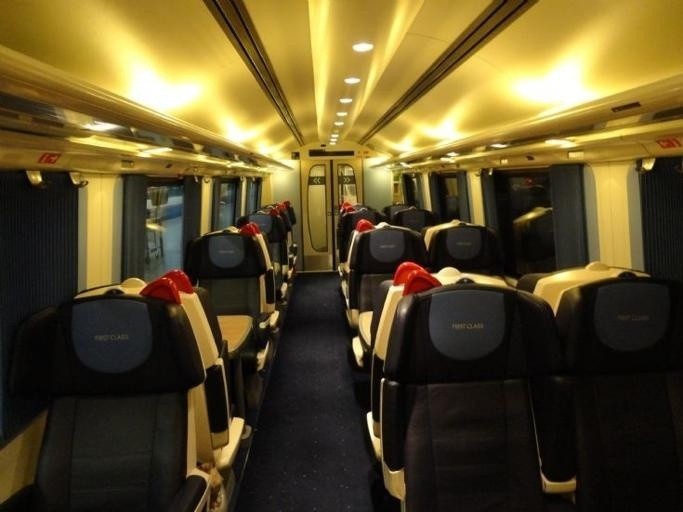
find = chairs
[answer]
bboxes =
[3,270,252,509]
[340,201,680,511]
[120,203,295,379]
